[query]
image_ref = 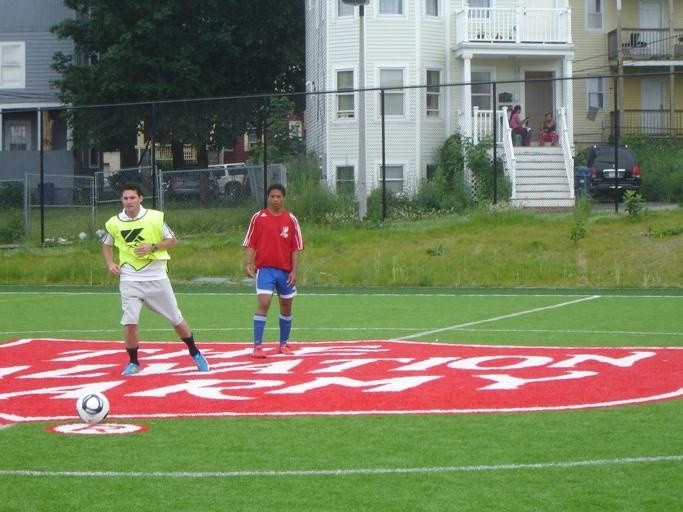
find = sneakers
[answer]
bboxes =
[279,345,295,355]
[121,363,140,376]
[252,346,266,357]
[190,350,209,372]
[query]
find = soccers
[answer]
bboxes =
[76,393,110,422]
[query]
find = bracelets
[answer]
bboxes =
[245,263,251,265]
[151,243,156,254]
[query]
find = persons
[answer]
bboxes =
[508,104,531,146]
[537,112,558,148]
[240,184,305,357]
[101,184,210,375]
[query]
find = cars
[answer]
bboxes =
[107,170,150,198]
[167,168,219,203]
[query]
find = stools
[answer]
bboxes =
[511,132,524,146]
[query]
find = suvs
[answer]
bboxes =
[574,141,640,200]
[206,163,248,199]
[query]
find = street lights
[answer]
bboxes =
[342,0,369,222]
